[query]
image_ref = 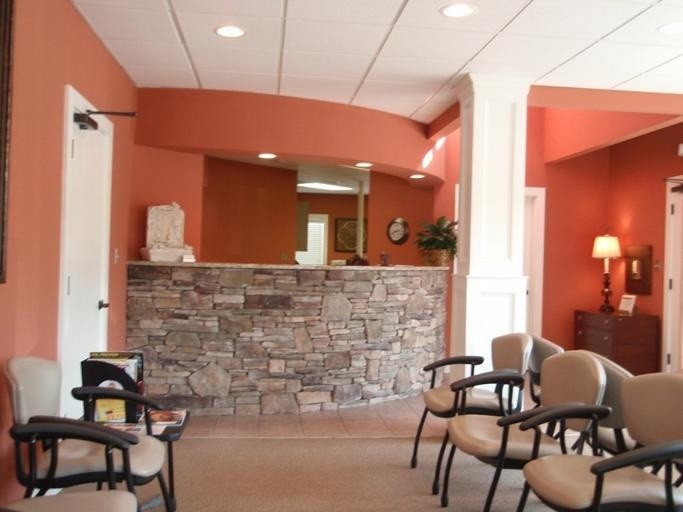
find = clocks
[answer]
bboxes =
[388,218,407,242]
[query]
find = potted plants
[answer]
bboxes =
[412,215,458,266]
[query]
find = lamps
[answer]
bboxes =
[590,234,622,313]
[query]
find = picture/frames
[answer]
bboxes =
[334,217,367,252]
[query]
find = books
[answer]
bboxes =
[87,351,143,423]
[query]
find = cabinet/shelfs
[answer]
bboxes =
[573,308,660,375]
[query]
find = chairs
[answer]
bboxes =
[0,356,190,511]
[410,333,535,495]
[516,373,682,510]
[441,351,612,510]
[508,334,682,485]
